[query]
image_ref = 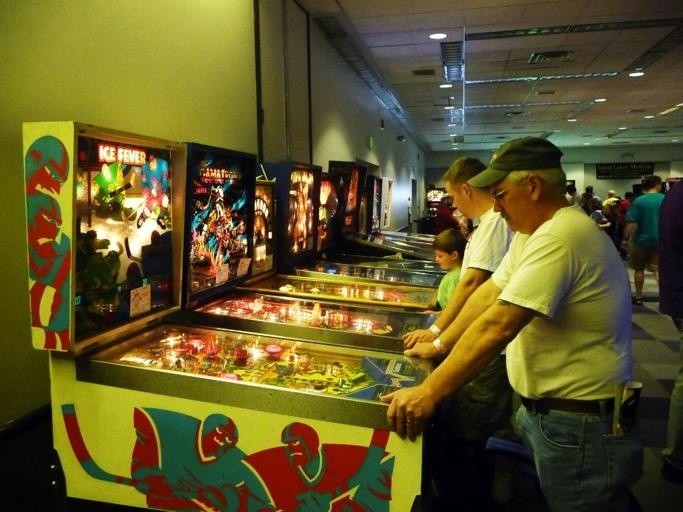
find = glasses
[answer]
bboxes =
[492,176,528,200]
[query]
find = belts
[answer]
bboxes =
[521,397,614,415]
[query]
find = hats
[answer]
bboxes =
[466,137,563,190]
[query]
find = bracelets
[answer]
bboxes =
[433,339,448,354]
[429,324,442,336]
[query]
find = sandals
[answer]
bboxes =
[632,296,643,304]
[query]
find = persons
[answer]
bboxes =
[658,178,682,480]
[565,176,667,304]
[418,227,465,316]
[380,136,644,512]
[400,157,541,512]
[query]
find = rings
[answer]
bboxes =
[405,418,412,424]
